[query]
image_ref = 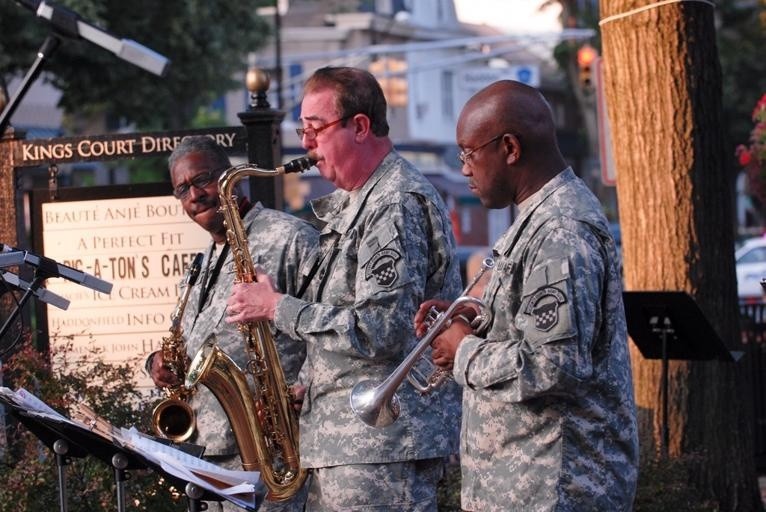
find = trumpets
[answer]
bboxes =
[350,258,495,430]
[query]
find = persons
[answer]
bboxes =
[146,134,321,511]
[224,69,465,511]
[412,78,639,511]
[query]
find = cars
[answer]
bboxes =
[736,240,766,305]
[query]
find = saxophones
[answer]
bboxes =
[185,157,313,503]
[152,253,203,442]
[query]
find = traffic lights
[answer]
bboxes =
[579,47,597,94]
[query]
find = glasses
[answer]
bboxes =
[173,164,229,201]
[456,135,507,167]
[294,113,356,143]
